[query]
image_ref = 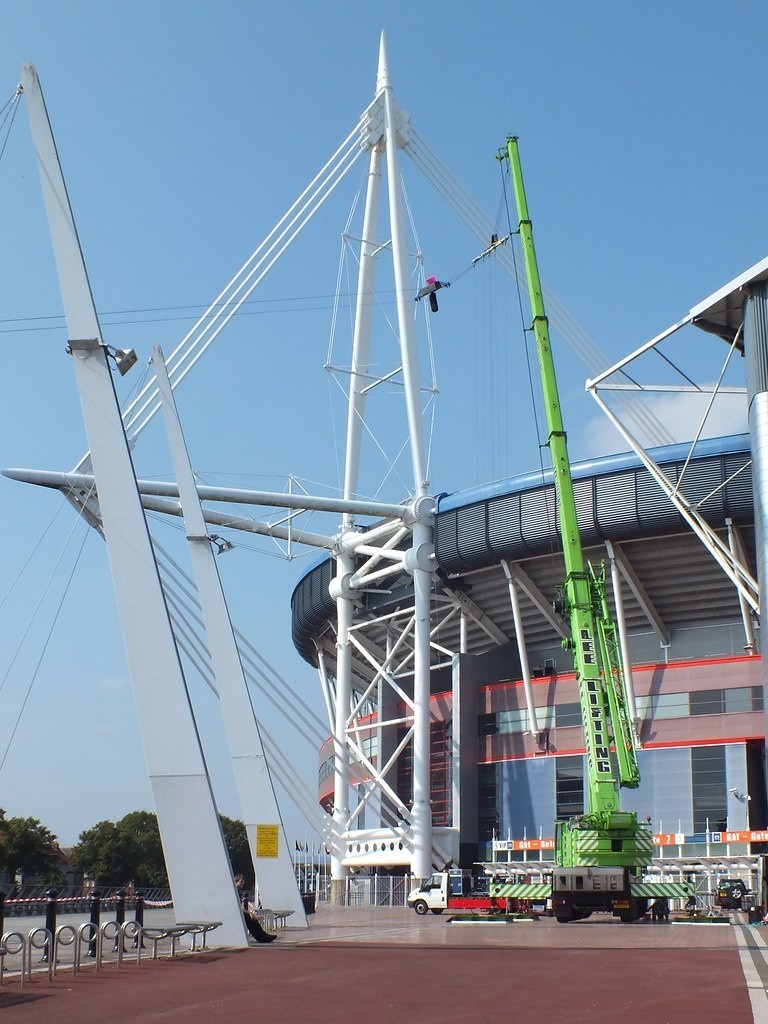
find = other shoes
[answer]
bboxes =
[260,933,278,945]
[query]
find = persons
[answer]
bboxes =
[234,874,277,944]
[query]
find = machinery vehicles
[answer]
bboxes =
[415,132,699,924]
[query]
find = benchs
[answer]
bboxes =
[130,925,198,958]
[176,919,224,951]
[271,909,296,931]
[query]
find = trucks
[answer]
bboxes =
[404,860,533,917]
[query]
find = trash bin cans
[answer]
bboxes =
[301,892,316,915]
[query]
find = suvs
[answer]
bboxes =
[712,878,748,909]
